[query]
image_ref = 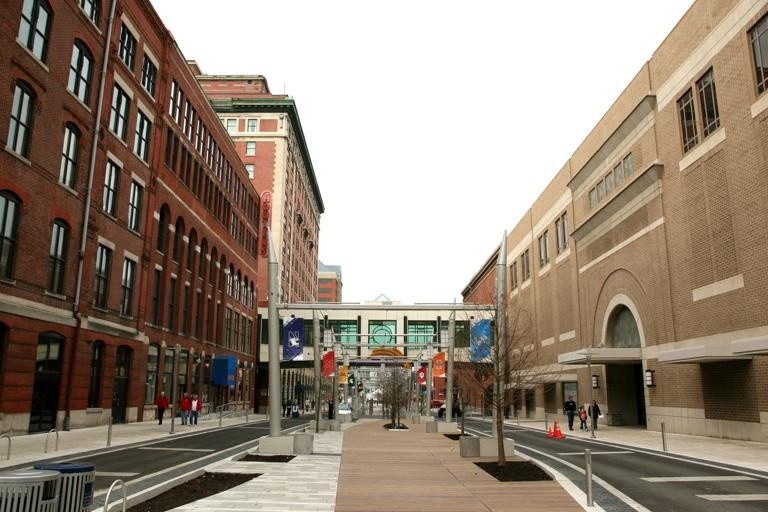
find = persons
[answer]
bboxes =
[577,404,589,432]
[563,395,577,430]
[189,392,203,425]
[281,397,330,419]
[154,390,169,424]
[587,399,602,430]
[179,391,192,425]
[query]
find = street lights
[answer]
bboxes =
[327,323,356,422]
[442,297,457,422]
[411,339,433,416]
[309,302,323,420]
[491,228,508,439]
[262,224,281,437]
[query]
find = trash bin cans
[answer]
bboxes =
[0,462,95,512]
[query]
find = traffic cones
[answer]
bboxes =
[547,427,552,438]
[553,418,558,438]
[555,421,566,440]
